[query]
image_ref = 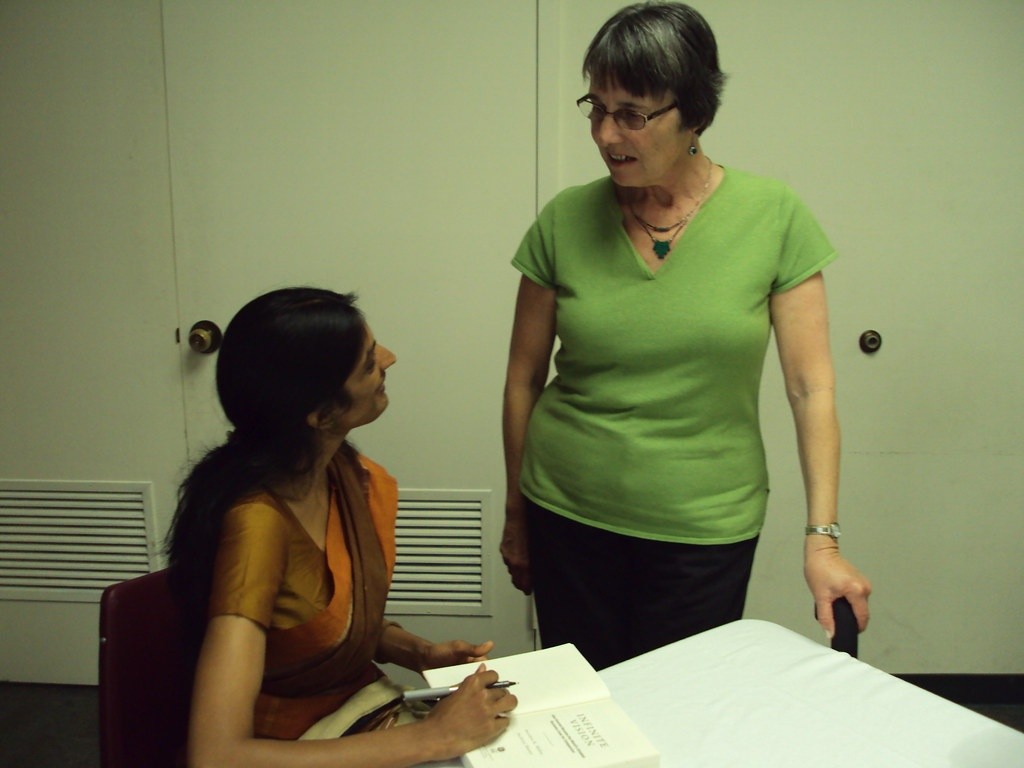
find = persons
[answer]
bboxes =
[165,288,517,768]
[498,0,871,673]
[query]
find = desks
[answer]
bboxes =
[454,619,1023,766]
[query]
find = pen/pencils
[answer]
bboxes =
[403,681,517,701]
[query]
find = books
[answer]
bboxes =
[421,643,661,768]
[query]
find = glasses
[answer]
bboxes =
[369,699,432,731]
[576,94,677,130]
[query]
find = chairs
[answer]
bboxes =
[97,567,197,767]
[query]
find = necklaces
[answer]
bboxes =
[628,154,712,259]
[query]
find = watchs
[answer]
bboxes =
[806,522,841,539]
